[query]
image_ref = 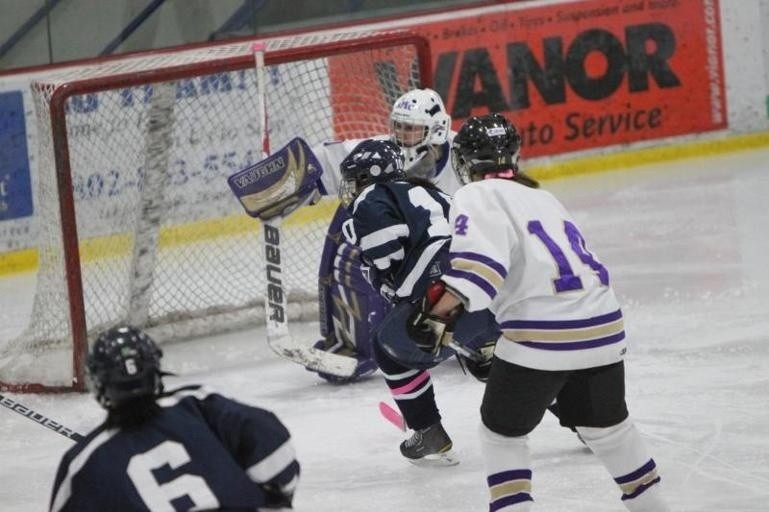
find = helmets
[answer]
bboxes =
[451,112,522,185]
[339,139,406,202]
[82,324,164,407]
[390,88,451,170]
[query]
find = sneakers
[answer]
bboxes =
[400,423,452,458]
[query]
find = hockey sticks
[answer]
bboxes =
[250,44,358,378]
[381,326,434,434]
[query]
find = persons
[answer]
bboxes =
[48,323,300,512]
[335,138,589,462]
[225,84,502,387]
[405,112,674,512]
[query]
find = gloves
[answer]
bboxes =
[466,338,496,383]
[407,294,447,357]
